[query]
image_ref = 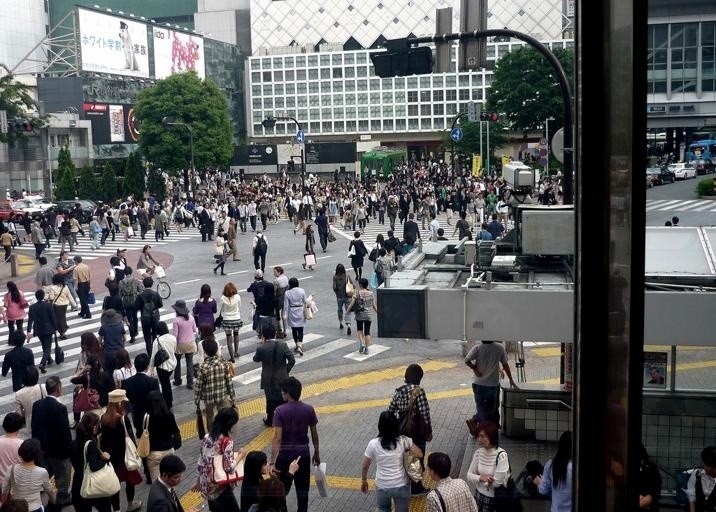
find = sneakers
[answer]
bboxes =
[364,347,371,355]
[347,325,351,338]
[339,323,344,332]
[39,363,50,374]
[359,344,367,353]
[172,379,184,387]
[186,383,197,390]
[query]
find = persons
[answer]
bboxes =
[217,171,237,217]
[123,353,160,428]
[671,216,679,226]
[235,166,282,232]
[1,330,34,392]
[69,355,114,407]
[466,421,511,512]
[8,219,17,247]
[135,277,163,358]
[149,321,177,408]
[387,363,433,497]
[647,367,664,385]
[0,412,24,493]
[218,211,231,252]
[73,254,92,319]
[31,376,72,479]
[27,289,58,374]
[190,407,246,512]
[282,169,313,235]
[346,277,377,356]
[171,299,198,390]
[106,256,131,331]
[69,412,120,512]
[56,251,77,306]
[348,232,368,281]
[464,340,520,437]
[536,168,563,205]
[313,173,326,216]
[102,283,124,313]
[172,168,217,243]
[665,221,672,226]
[112,348,136,389]
[282,277,308,356]
[533,430,573,512]
[213,227,227,276]
[253,323,296,427]
[35,257,55,290]
[4,281,29,335]
[0,227,14,262]
[314,210,329,252]
[333,264,356,335]
[326,169,378,241]
[194,339,238,435]
[240,450,301,512]
[1,438,58,512]
[272,266,289,339]
[113,193,172,243]
[192,284,217,328]
[15,365,47,439]
[0,218,4,234]
[69,332,103,429]
[445,164,509,241]
[136,390,181,483]
[247,269,276,317]
[43,273,77,340]
[100,388,143,512]
[118,266,143,343]
[378,156,445,241]
[270,375,321,512]
[427,452,479,512]
[368,241,418,289]
[361,411,424,512]
[7,212,22,246]
[97,308,127,373]
[679,445,716,512]
[22,189,27,198]
[218,282,243,363]
[136,244,163,278]
[56,200,113,251]
[197,322,221,368]
[22,205,56,259]
[116,248,127,267]
[252,229,269,274]
[302,224,317,270]
[224,218,241,261]
[639,443,663,512]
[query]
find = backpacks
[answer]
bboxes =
[120,279,140,310]
[141,296,159,326]
[256,235,267,256]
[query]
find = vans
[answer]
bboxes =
[1,203,43,224]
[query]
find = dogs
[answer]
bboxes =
[118,21,143,70]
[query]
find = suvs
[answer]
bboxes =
[16,199,56,214]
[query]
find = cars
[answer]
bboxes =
[56,200,105,222]
[691,158,716,175]
[670,162,697,180]
[647,166,675,186]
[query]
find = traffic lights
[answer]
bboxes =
[7,119,34,132]
[286,160,294,173]
[262,120,276,128]
[10,119,34,131]
[287,160,294,171]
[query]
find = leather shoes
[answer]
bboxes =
[126,500,144,511]
[263,419,272,426]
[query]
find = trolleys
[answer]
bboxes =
[339,209,354,230]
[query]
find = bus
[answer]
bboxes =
[686,139,716,161]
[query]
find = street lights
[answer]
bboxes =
[260,113,305,197]
[162,117,195,179]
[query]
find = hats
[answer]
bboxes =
[107,389,132,406]
[102,308,124,330]
[170,299,190,320]
[254,268,264,278]
[115,247,128,256]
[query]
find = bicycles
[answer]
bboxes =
[132,265,179,299]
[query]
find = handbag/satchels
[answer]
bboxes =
[369,244,383,261]
[73,371,101,412]
[404,439,422,484]
[347,245,357,260]
[495,450,527,512]
[120,416,142,469]
[195,404,205,442]
[120,217,128,226]
[92,224,104,233]
[87,290,96,305]
[352,291,364,314]
[53,332,65,366]
[127,228,135,238]
[18,294,28,310]
[43,225,54,234]
[327,229,336,243]
[302,251,316,268]
[0,466,29,511]
[345,277,355,296]
[212,243,226,258]
[80,441,120,497]
[104,276,118,294]
[209,437,245,483]
[304,303,315,321]
[399,387,430,440]
[215,315,224,329]
[154,336,169,367]
[137,414,152,459]
[312,464,330,499]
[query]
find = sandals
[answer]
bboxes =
[296,347,304,356]
[467,416,478,438]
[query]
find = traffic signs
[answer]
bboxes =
[446,130,467,145]
[292,130,310,143]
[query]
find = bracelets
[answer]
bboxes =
[269,462,274,466]
